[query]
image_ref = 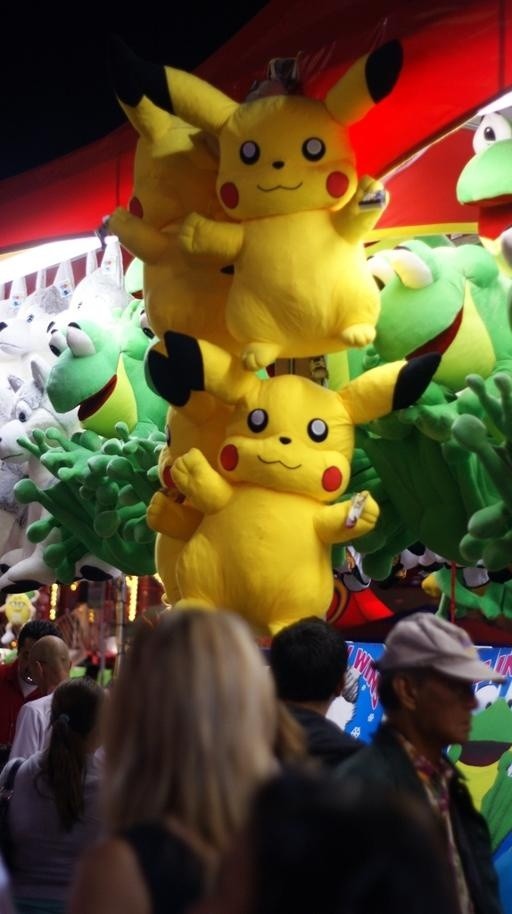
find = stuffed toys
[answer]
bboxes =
[2,259,82,353]
[144,392,236,607]
[157,327,441,639]
[365,243,512,578]
[8,324,137,572]
[2,363,83,588]
[84,329,171,575]
[165,39,406,370]
[457,109,512,207]
[102,75,249,364]
[340,342,408,580]
[15,322,147,578]
[63,235,124,322]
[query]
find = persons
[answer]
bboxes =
[328,611,507,914]
[2,679,106,914]
[70,601,322,914]
[0,618,67,768]
[0,634,72,802]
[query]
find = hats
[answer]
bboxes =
[371,613,506,681]
